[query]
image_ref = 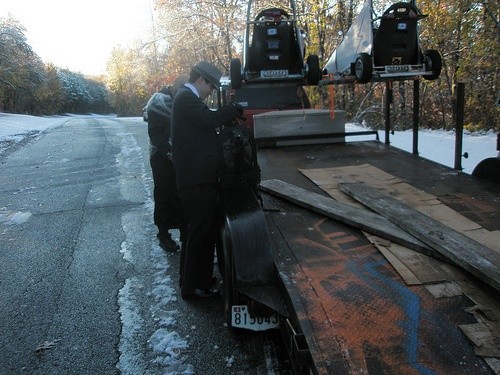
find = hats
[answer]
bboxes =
[191,61,222,91]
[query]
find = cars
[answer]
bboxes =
[142,107,149,121]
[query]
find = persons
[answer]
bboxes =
[172,61,246,301]
[147,75,189,252]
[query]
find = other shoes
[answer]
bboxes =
[156,232,180,252]
[177,276,222,300]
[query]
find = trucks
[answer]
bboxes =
[218,108,500,375]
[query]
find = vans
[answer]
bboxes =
[210,76,314,148]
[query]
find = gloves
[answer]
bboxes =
[233,103,244,117]
